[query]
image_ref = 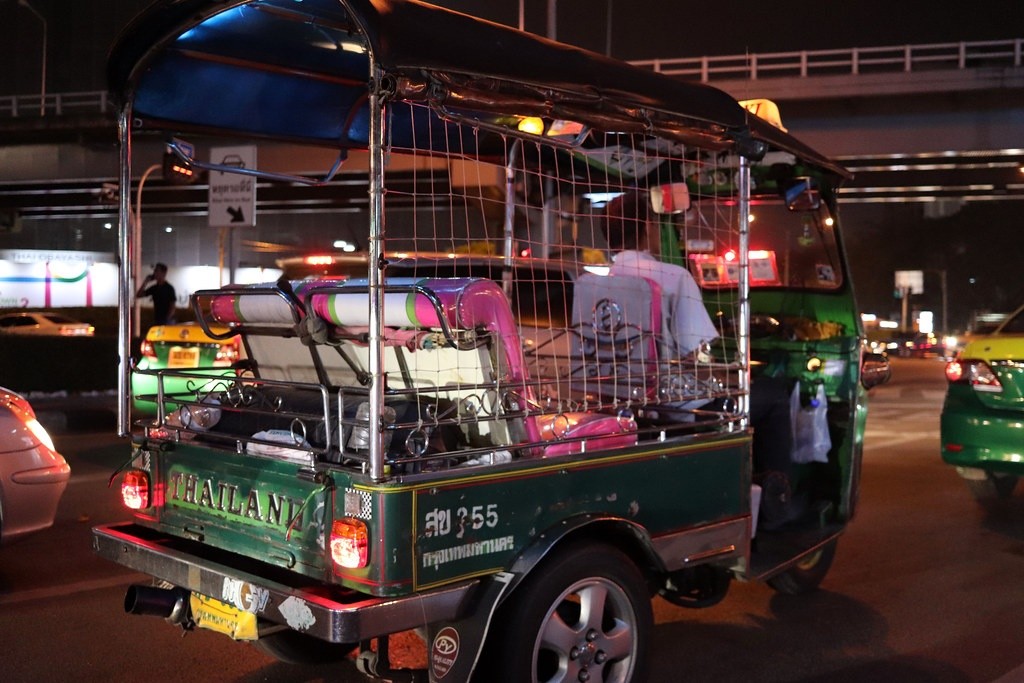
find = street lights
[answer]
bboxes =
[18,0,48,116]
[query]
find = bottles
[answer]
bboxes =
[147,400,221,439]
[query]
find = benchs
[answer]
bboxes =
[207,276,638,461]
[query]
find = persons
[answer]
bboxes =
[137,263,177,326]
[600,191,810,532]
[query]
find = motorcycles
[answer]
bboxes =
[94,1,892,683]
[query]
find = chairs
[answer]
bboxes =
[569,272,693,440]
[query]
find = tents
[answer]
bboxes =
[118,0,760,487]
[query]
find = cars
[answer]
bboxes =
[0,312,96,337]
[0,386,72,548]
[939,304,1024,504]
[914,334,944,358]
